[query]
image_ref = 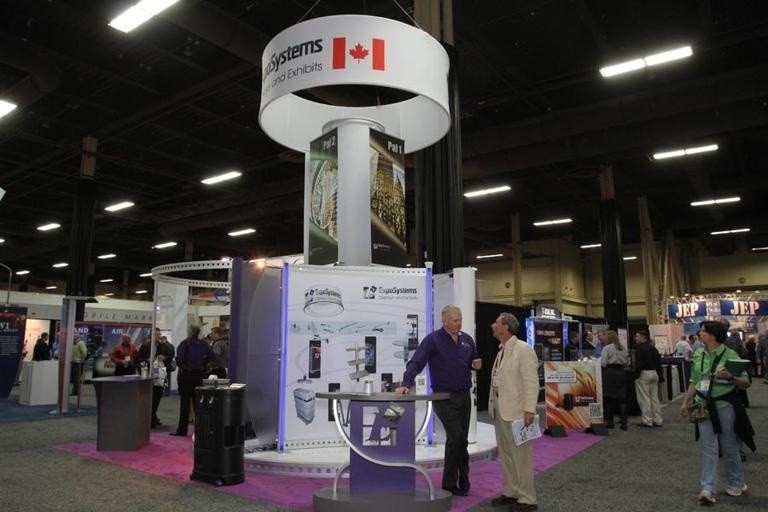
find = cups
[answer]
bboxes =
[141,366,149,378]
[363,379,373,394]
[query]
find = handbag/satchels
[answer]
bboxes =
[689,401,707,423]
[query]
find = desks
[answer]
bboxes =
[309,389,455,512]
[80,374,160,452]
[188,383,248,486]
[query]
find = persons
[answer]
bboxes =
[15,323,229,437]
[487,313,540,512]
[395,304,483,497]
[564,317,768,504]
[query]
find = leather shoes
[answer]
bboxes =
[493,495,537,512]
[442,467,470,496]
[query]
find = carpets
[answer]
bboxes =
[0,394,99,425]
[53,425,608,512]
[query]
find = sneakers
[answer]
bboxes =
[724,483,747,496]
[698,489,716,503]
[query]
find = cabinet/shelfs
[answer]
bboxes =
[18,360,61,406]
